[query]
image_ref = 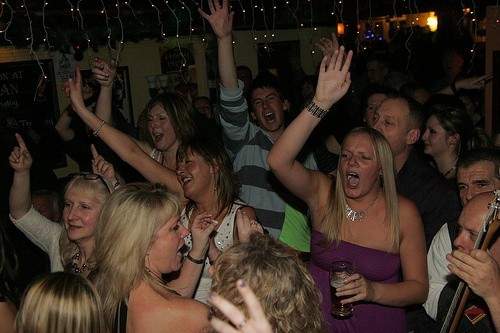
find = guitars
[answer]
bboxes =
[439,188,500,333]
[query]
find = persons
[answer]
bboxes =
[436,189,500,333]
[264,45,429,333]
[173,134,264,301]
[58,66,271,309]
[86,182,217,333]
[6,126,116,278]
[249,221,258,225]
[0,0,500,333]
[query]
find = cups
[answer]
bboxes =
[329,260,354,316]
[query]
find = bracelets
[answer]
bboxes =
[91,121,108,136]
[307,100,328,120]
[113,178,123,188]
[187,250,206,264]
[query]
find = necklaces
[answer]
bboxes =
[343,190,378,223]
[442,154,458,178]
[72,253,91,276]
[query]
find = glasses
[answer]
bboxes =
[66,173,111,194]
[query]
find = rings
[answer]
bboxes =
[196,0,317,258]
[329,45,333,48]
[236,317,248,331]
[91,67,96,73]
